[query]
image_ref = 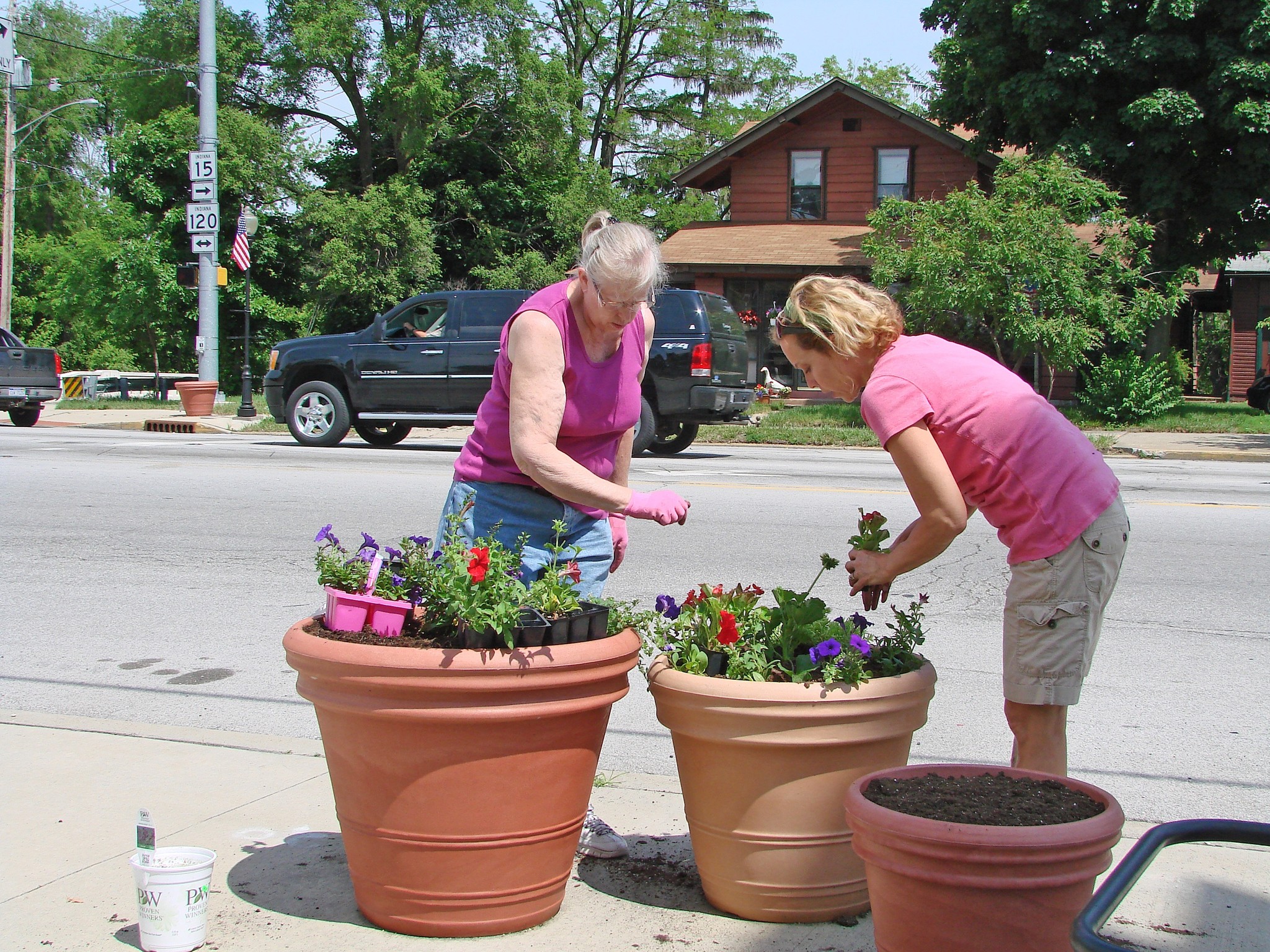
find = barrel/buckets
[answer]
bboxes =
[130,846,217,952]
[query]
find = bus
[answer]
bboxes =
[58,368,199,402]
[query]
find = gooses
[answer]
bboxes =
[761,365,785,390]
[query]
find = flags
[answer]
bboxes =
[231,209,250,271]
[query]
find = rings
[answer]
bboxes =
[849,572,855,581]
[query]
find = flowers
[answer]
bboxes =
[781,386,792,394]
[319,528,581,651]
[738,308,762,327]
[753,379,773,398]
[766,307,782,319]
[656,504,936,691]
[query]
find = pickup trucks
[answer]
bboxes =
[0,327,61,427]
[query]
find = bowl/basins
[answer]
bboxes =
[175,381,219,416]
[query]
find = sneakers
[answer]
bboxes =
[575,803,628,858]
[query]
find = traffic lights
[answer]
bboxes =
[175,266,198,287]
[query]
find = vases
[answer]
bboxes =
[281,588,1126,951]
[174,381,220,415]
[741,322,758,332]
[783,393,791,399]
[769,318,777,327]
[758,394,770,404]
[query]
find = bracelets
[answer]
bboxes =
[411,326,417,332]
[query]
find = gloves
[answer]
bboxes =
[622,490,691,526]
[608,512,628,573]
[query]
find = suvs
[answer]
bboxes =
[263,290,755,457]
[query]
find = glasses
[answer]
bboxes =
[775,310,832,338]
[592,279,656,310]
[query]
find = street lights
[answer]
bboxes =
[1,96,100,331]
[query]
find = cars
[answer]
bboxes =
[1245,376,1270,413]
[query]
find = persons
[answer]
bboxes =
[403,322,445,338]
[433,212,690,602]
[777,275,1131,779]
